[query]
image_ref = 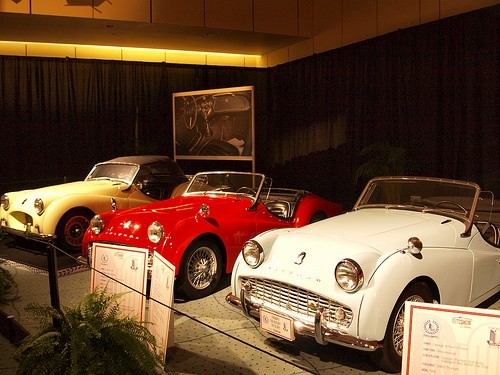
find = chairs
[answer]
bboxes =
[263,200,291,217]
[144,187,162,200]
[476,222,497,247]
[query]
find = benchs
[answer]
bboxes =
[256,188,303,216]
[422,196,500,234]
[140,173,181,197]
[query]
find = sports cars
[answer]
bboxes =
[82,171,345,299]
[4,156,216,255]
[225,175,500,373]
